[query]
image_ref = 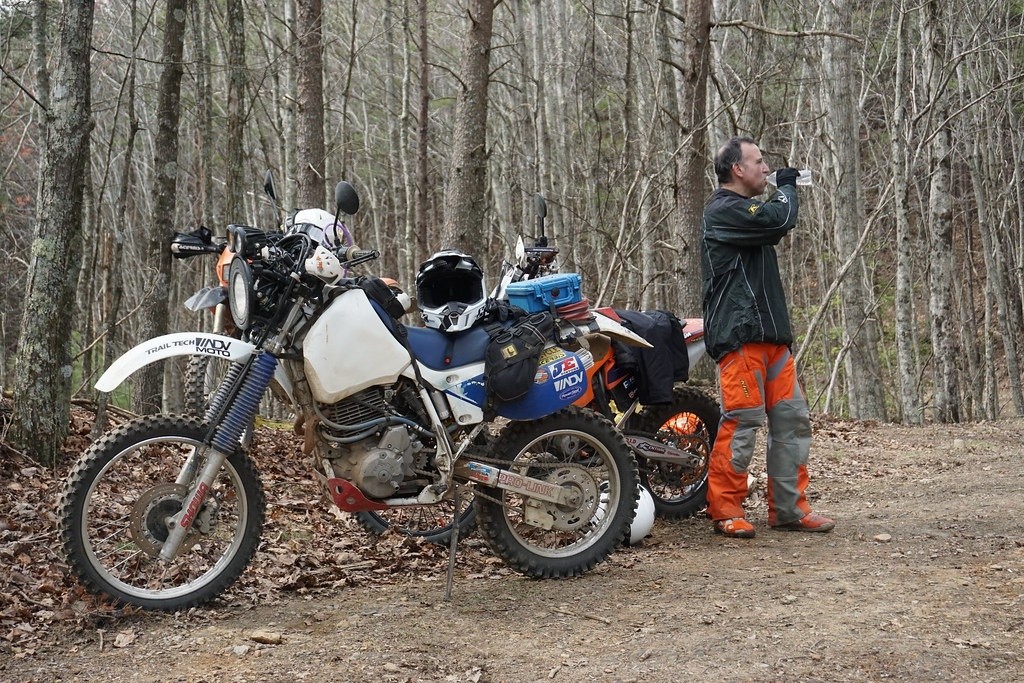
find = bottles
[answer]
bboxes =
[765,170,812,186]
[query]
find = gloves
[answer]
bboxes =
[484,297,521,325]
[776,167,800,188]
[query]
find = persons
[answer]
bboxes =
[700,136,836,538]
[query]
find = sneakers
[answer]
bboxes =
[713,517,756,538]
[765,509,836,531]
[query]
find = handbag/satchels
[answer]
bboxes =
[481,310,556,409]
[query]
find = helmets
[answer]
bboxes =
[415,247,488,332]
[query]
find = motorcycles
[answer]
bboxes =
[328,193,722,553]
[56,180,640,613]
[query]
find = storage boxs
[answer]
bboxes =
[504,272,582,312]
